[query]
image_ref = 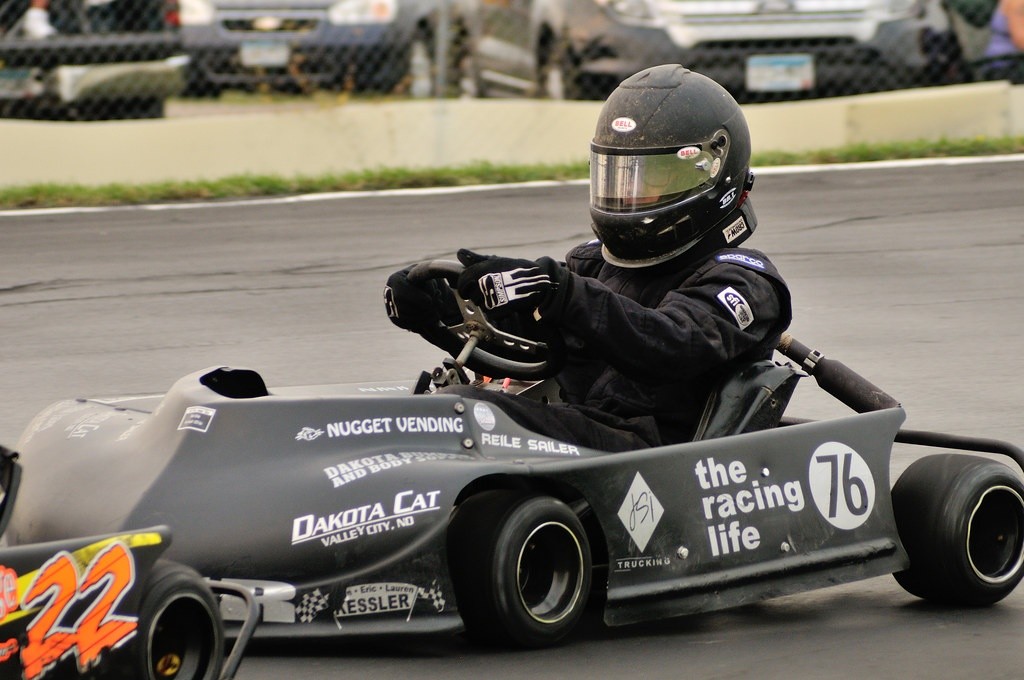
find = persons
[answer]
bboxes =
[983,1,1024,84]
[23,0,180,117]
[384,64,792,452]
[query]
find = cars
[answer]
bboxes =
[178,1,424,100]
[450,2,970,106]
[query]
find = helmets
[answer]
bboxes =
[590,62,755,259]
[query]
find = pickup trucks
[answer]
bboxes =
[1,1,193,121]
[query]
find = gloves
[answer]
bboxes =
[383,260,454,342]
[449,248,574,333]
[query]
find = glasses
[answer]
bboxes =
[596,154,672,189]
